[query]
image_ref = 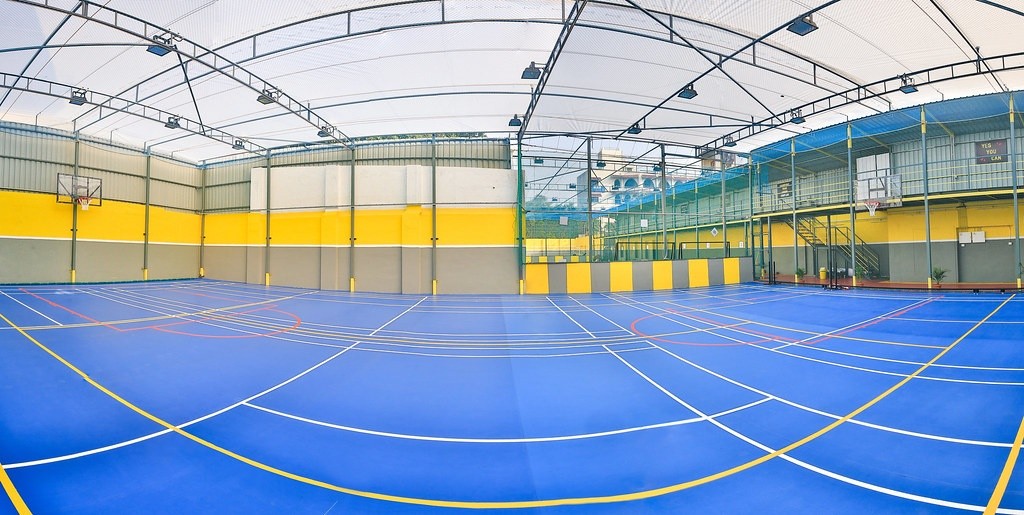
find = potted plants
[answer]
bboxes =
[933,267,952,288]
[797,268,807,283]
[855,267,870,286]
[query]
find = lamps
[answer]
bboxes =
[725,137,737,149]
[653,187,661,193]
[790,112,806,124]
[146,35,172,58]
[256,89,276,106]
[232,140,245,151]
[521,61,546,79]
[69,91,88,106]
[524,183,619,211]
[534,158,544,164]
[317,126,331,138]
[596,161,607,168]
[652,165,661,172]
[677,84,698,100]
[628,124,642,135]
[899,78,918,94]
[786,13,819,37]
[701,170,711,176]
[508,115,526,126]
[165,116,180,130]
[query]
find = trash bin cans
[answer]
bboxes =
[761,269,766,279]
[819,267,827,279]
